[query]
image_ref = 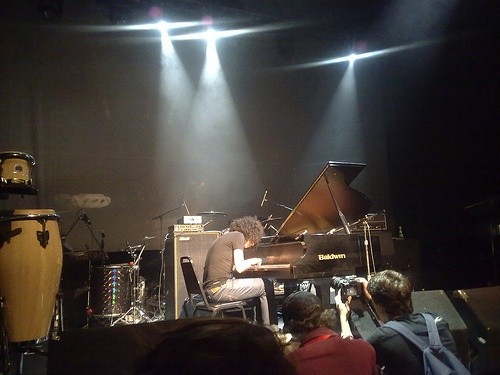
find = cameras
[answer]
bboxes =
[329,275,364,303]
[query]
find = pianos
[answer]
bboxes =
[231,161,411,325]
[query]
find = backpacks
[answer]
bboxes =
[384,312,470,375]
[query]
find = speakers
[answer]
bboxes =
[163,231,224,323]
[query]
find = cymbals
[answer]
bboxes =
[137,236,158,243]
[121,244,142,251]
[72,193,111,210]
[196,211,229,218]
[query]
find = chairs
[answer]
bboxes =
[180,256,248,319]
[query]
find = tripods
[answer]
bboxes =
[110,248,153,327]
[79,220,106,328]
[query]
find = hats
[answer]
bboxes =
[282,293,321,334]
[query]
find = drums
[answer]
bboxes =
[66,249,107,293]
[1,208,64,344]
[0,151,38,194]
[89,263,137,319]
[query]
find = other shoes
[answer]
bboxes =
[275,331,293,345]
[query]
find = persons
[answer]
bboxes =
[203,216,292,343]
[282,292,378,375]
[334,270,457,375]
[132,316,297,375]
